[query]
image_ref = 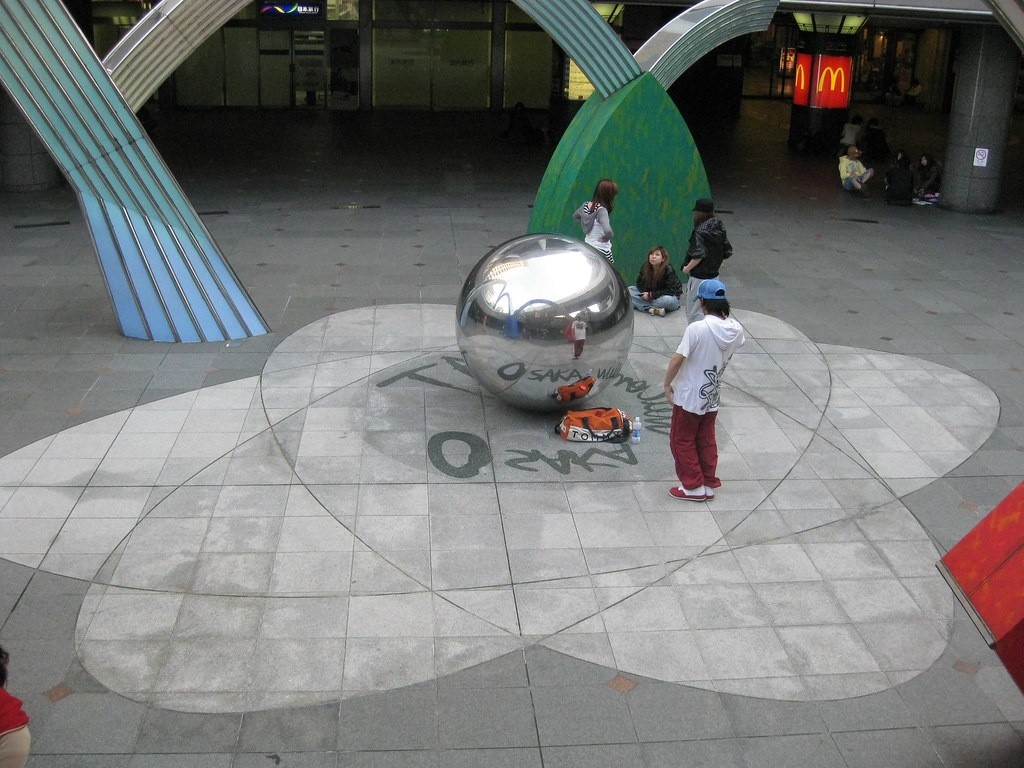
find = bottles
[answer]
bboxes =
[632,417,641,444]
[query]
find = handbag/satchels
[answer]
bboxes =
[552,376,598,402]
[554,407,633,443]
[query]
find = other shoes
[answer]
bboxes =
[572,357,576,360]
[668,485,707,502]
[649,306,665,317]
[577,354,582,358]
[705,485,715,501]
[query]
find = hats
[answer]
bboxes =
[693,279,726,302]
[692,198,714,212]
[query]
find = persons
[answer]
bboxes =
[571,314,588,359]
[680,198,733,326]
[663,278,745,501]
[838,114,942,205]
[507,102,532,136]
[574,179,618,264]
[903,79,923,104]
[628,246,682,317]
